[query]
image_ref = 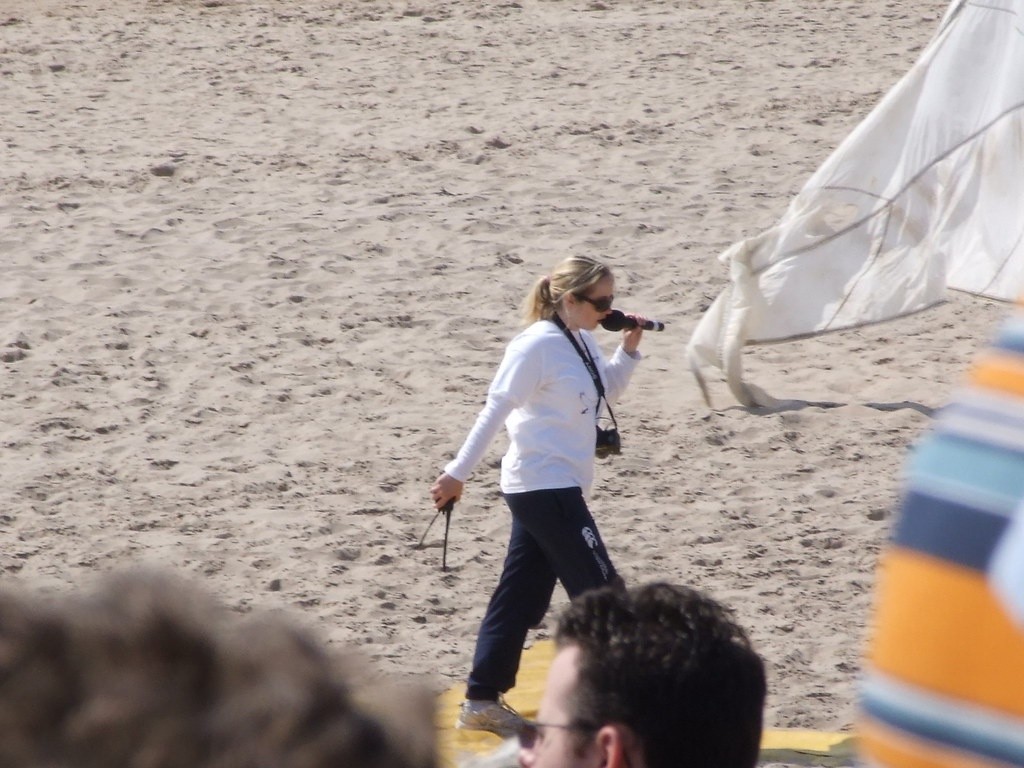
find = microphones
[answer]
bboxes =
[602,310,665,332]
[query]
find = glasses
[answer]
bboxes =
[517,721,632,768]
[572,292,614,313]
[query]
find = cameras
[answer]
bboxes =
[593,425,621,459]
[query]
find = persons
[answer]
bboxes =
[517,581,767,768]
[429,255,645,739]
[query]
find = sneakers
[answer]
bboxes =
[455,691,537,738]
